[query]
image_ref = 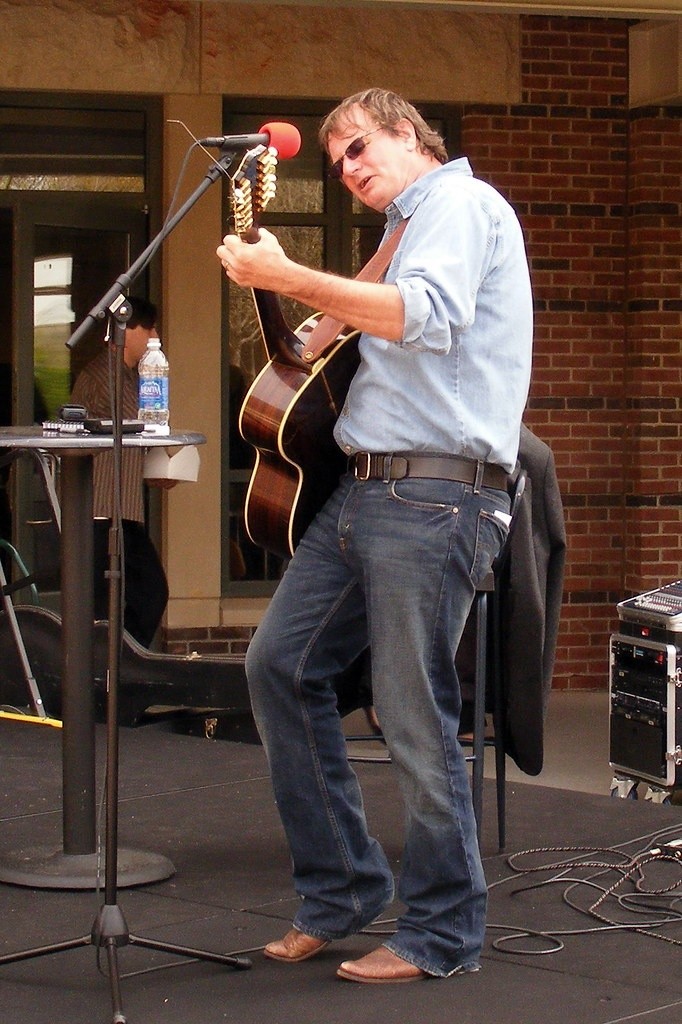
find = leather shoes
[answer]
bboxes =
[264,928,328,961]
[337,944,464,983]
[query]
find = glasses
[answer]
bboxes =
[329,127,385,178]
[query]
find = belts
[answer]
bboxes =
[347,453,507,492]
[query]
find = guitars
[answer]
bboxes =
[224,145,363,561]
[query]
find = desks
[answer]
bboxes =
[1,426,205,889]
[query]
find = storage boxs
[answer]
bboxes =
[144,445,200,482]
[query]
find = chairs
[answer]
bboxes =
[341,469,528,849]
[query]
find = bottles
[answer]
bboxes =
[138,338,168,436]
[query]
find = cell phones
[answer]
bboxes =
[60,404,87,419]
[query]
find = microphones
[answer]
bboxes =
[197,123,302,159]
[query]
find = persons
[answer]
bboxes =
[55,298,179,650]
[215,87,533,984]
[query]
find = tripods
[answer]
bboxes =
[0,147,252,1024]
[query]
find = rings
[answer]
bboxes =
[224,263,230,271]
